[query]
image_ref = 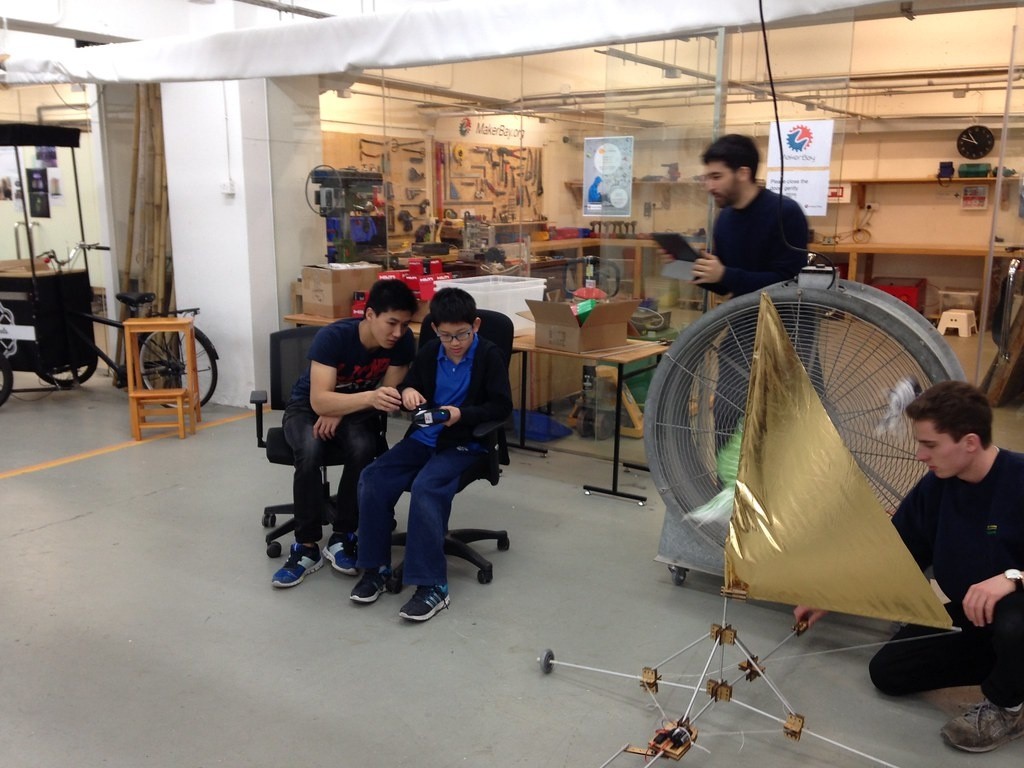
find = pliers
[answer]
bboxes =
[506,147,527,161]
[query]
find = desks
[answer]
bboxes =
[388,238,1024,322]
[511,333,670,506]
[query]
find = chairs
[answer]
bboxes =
[248,325,397,560]
[391,309,511,586]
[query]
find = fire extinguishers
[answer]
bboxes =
[562,256,620,301]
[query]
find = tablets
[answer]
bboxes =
[651,231,703,264]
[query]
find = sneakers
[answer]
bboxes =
[939,698,1024,752]
[271,541,324,588]
[321,532,359,576]
[399,582,451,621]
[350,564,393,603]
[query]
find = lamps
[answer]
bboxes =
[70,83,86,93]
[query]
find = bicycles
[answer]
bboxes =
[35,241,220,410]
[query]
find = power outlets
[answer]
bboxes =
[652,201,662,209]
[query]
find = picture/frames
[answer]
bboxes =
[30,192,50,218]
[25,167,48,192]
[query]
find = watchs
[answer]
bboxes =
[1004,569,1024,594]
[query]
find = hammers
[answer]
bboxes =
[503,159,510,188]
[510,165,516,188]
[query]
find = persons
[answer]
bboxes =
[272,278,420,589]
[349,286,514,621]
[654,132,810,486]
[791,380,1024,752]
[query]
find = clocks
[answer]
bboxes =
[957,125,995,160]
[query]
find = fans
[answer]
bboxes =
[642,262,968,559]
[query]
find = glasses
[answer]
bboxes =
[435,320,473,342]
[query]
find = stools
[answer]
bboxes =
[131,388,188,441]
[121,317,202,435]
[937,308,978,337]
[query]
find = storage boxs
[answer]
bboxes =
[872,276,927,315]
[405,275,418,291]
[577,228,590,238]
[412,241,450,256]
[958,163,991,179]
[353,290,370,304]
[631,310,671,331]
[430,285,548,333]
[558,227,580,239]
[429,259,444,275]
[409,258,424,275]
[432,275,548,289]
[302,261,384,320]
[419,272,453,301]
[936,289,981,317]
[514,299,640,354]
[350,303,371,318]
[378,269,410,287]
